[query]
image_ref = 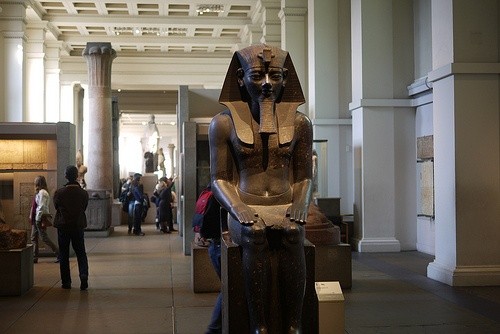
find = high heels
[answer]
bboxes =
[160,228,171,233]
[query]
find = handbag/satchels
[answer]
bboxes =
[40,213,53,227]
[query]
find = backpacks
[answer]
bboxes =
[191,183,221,247]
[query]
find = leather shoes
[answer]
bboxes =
[62,284,72,289]
[54,258,60,263]
[80,281,89,290]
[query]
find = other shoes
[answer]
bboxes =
[128,224,144,236]
[169,227,179,232]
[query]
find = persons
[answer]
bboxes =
[128,173,145,237]
[209,44,314,334]
[26,174,61,264]
[152,177,179,233]
[190,189,222,333]
[119,176,133,226]
[52,166,90,290]
